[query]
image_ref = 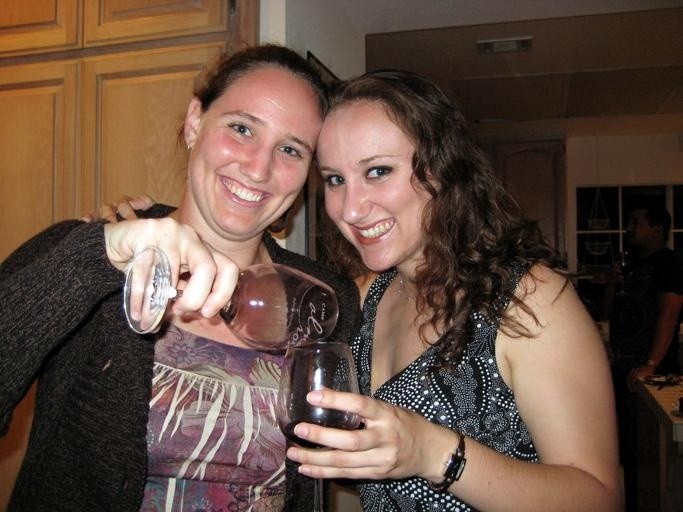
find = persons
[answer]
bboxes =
[610,196,681,511]
[82,63,628,512]
[2,38,363,511]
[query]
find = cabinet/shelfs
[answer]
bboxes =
[1,1,260,510]
[487,120,566,274]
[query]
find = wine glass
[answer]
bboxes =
[615,250,634,297]
[276,340,361,512]
[124,244,339,351]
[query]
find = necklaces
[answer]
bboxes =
[393,275,439,302]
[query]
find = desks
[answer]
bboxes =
[631,372,682,511]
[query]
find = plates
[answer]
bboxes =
[644,375,681,386]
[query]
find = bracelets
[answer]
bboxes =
[644,359,658,369]
[431,432,468,494]
